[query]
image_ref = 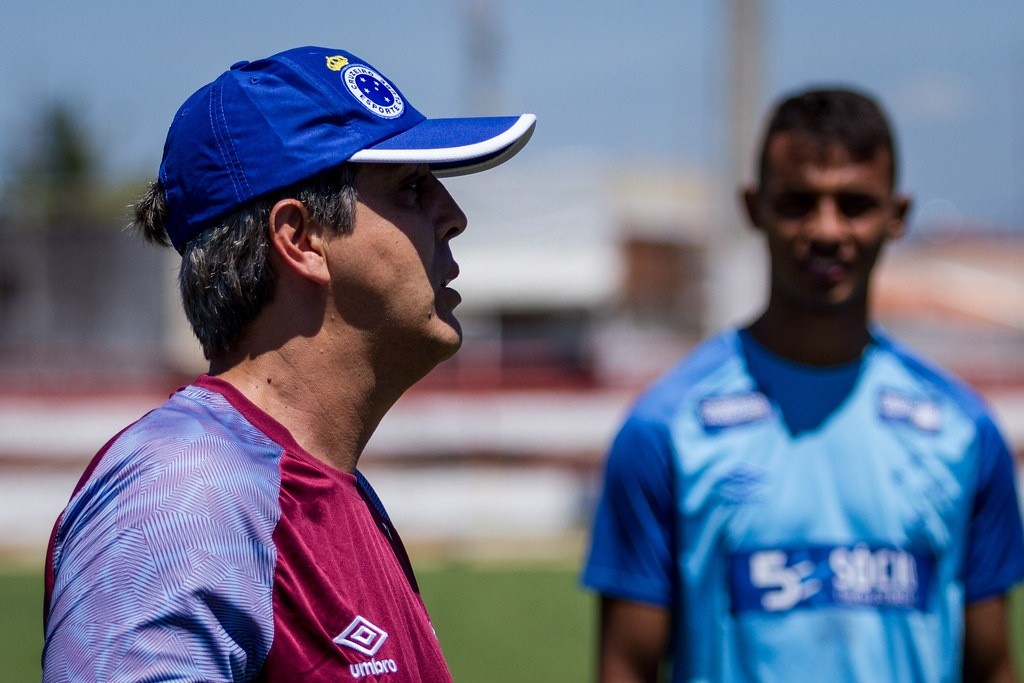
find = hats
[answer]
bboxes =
[157,46,537,256]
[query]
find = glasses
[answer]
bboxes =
[758,185,884,221]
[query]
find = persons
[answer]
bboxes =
[579,86,1024,683]
[39,48,536,682]
